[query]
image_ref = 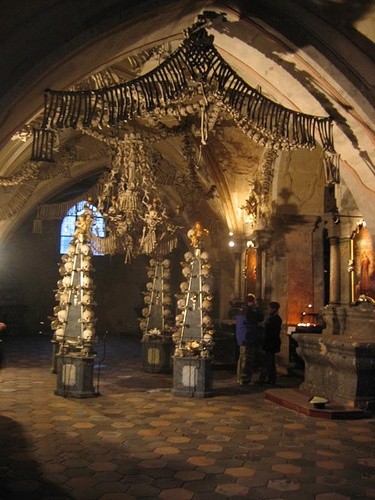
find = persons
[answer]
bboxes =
[253,297,264,322]
[236,293,256,385]
[253,302,281,387]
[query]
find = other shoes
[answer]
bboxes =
[239,380,255,386]
[254,381,262,386]
[262,382,275,387]
